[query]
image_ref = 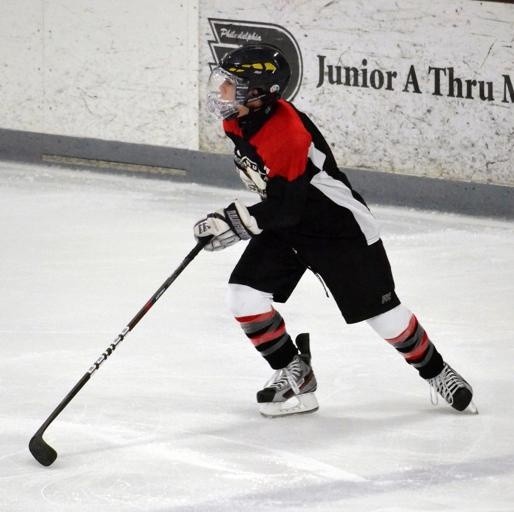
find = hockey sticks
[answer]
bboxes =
[29,235,214,467]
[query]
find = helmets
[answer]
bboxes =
[207,44,290,120]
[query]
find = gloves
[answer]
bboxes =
[193,199,263,252]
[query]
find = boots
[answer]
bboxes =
[257,354,317,402]
[424,361,473,411]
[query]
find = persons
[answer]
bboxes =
[190,44,479,415]
